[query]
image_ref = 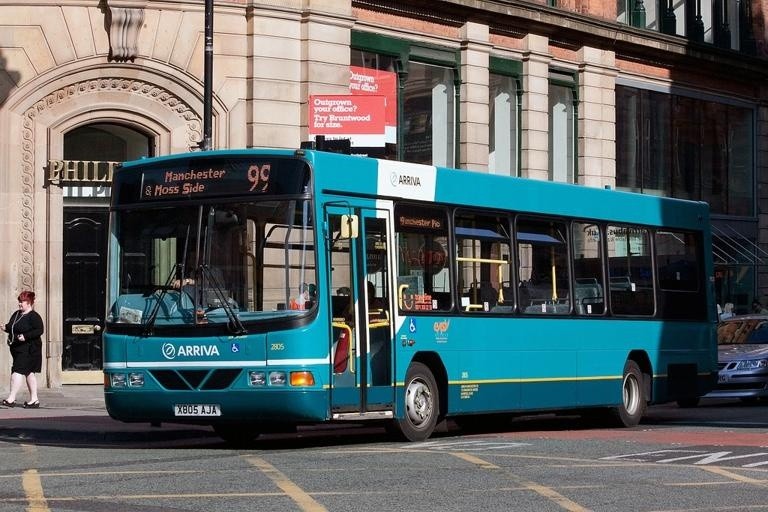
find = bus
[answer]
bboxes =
[101,148,719,444]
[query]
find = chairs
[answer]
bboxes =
[298,264,700,316]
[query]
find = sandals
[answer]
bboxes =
[2,399,16,408]
[23,399,40,408]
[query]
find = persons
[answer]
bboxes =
[292,282,310,310]
[342,280,388,362]
[170,245,228,292]
[0,290,44,407]
[750,299,768,315]
[718,302,736,322]
[306,283,318,310]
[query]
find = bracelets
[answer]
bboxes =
[186,277,191,285]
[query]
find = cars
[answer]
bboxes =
[676,314,768,408]
